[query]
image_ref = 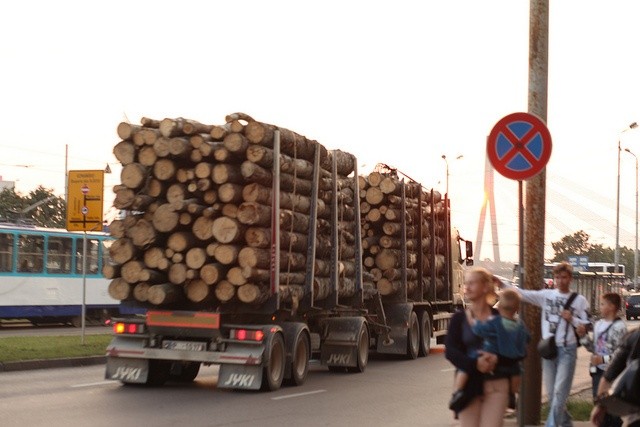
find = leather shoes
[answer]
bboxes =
[449,390,467,411]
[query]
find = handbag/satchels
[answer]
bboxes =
[593,358,640,415]
[537,336,558,358]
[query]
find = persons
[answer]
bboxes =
[589,325,640,427]
[444,267,517,426]
[503,263,591,426]
[576,292,628,405]
[450,287,532,412]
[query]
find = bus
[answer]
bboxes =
[0,222,119,326]
[511,261,625,289]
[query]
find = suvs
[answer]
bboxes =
[623,293,640,320]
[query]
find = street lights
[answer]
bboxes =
[625,148,638,279]
[441,154,463,194]
[614,120,640,271]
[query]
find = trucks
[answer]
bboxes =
[104,112,474,391]
